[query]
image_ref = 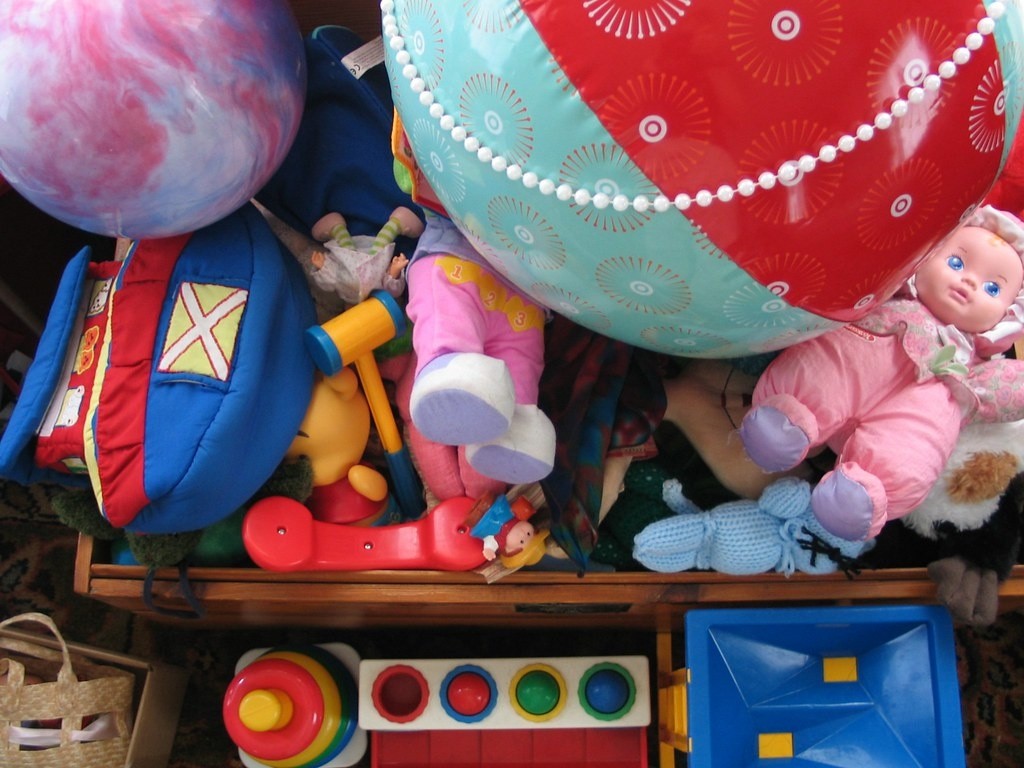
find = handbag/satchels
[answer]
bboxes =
[0,612,136,768]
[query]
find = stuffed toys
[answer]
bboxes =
[43,312,1024,626]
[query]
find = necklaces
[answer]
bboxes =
[379,0,1006,212]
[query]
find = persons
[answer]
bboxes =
[309,207,424,312]
[405,209,556,485]
[741,204,1024,543]
[463,490,550,568]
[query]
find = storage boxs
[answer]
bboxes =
[0,625,189,768]
[73,238,1024,636]
